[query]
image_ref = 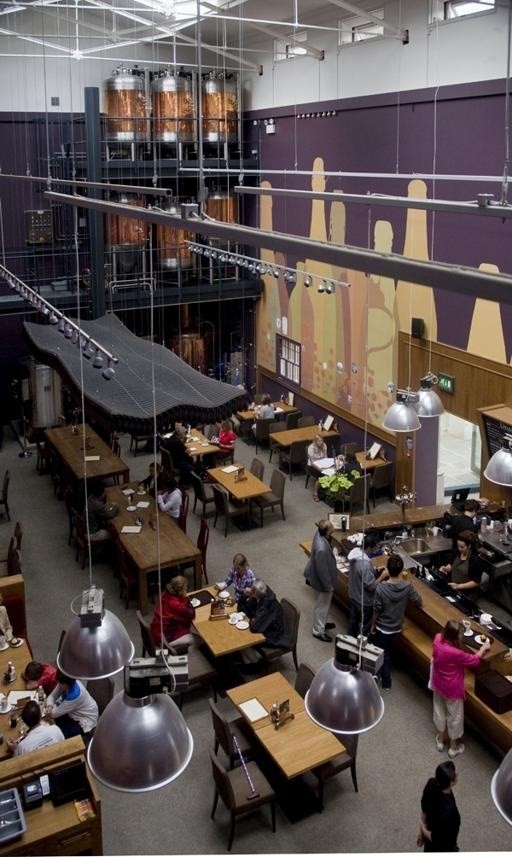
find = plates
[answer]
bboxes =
[481,613,493,624]
[463,629,474,636]
[1,642,9,651]
[190,588,250,630]
[136,490,147,495]
[126,504,136,511]
[186,433,209,450]
[0,703,12,715]
[474,633,490,645]
[10,637,24,648]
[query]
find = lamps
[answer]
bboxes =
[87,220,194,793]
[56,205,135,681]
[184,239,351,295]
[304,272,385,735]
[381,279,421,433]
[0,266,118,380]
[484,433,512,487]
[414,286,444,417]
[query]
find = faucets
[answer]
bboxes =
[393,535,410,546]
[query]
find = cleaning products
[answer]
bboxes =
[402,528,408,540]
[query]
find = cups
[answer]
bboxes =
[0,635,6,648]
[463,620,472,633]
[137,485,144,493]
[0,732,5,744]
[0,696,8,710]
[432,526,439,536]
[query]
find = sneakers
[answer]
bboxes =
[447,741,465,759]
[313,493,320,501]
[434,732,446,752]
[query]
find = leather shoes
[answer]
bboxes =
[313,632,332,642]
[325,622,335,629]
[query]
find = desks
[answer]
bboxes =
[45,423,130,508]
[225,672,347,824]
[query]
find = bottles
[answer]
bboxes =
[480,516,487,533]
[341,516,347,532]
[234,468,239,483]
[4,660,17,684]
[414,565,426,579]
[37,685,46,704]
[128,494,132,505]
[318,419,323,431]
[9,707,19,728]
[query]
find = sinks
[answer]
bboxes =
[396,538,433,556]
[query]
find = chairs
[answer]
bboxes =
[0,521,23,577]
[51,448,76,502]
[95,418,121,486]
[305,442,393,517]
[137,573,301,712]
[210,749,277,852]
[294,663,317,699]
[191,458,288,538]
[237,400,341,481]
[157,417,234,484]
[310,732,359,815]
[208,698,260,771]
[86,678,114,716]
[0,469,11,522]
[35,434,48,476]
